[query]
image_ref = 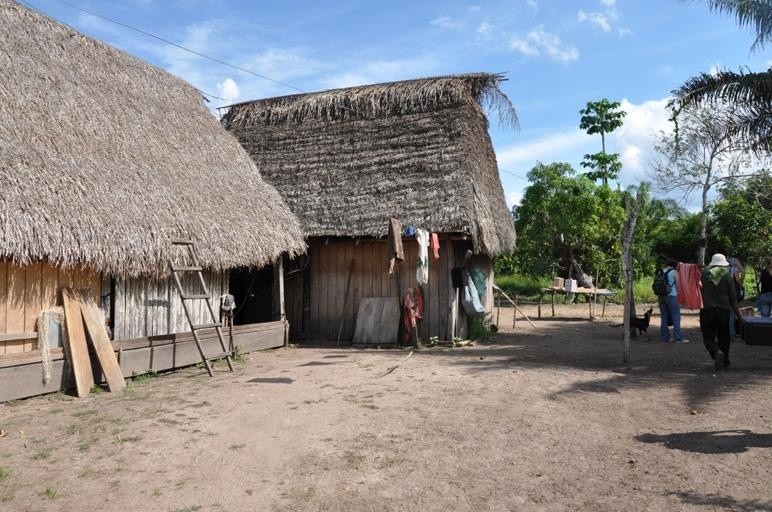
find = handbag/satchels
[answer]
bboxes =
[733,274,745,303]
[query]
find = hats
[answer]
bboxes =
[708,253,730,267]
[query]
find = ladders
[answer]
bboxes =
[166,241,234,376]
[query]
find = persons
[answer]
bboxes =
[652,252,771,371]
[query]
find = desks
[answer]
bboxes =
[535,287,618,319]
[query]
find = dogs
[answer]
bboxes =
[608,308,653,341]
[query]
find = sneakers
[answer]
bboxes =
[714,349,725,370]
[676,338,690,343]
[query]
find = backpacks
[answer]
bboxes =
[651,269,674,296]
